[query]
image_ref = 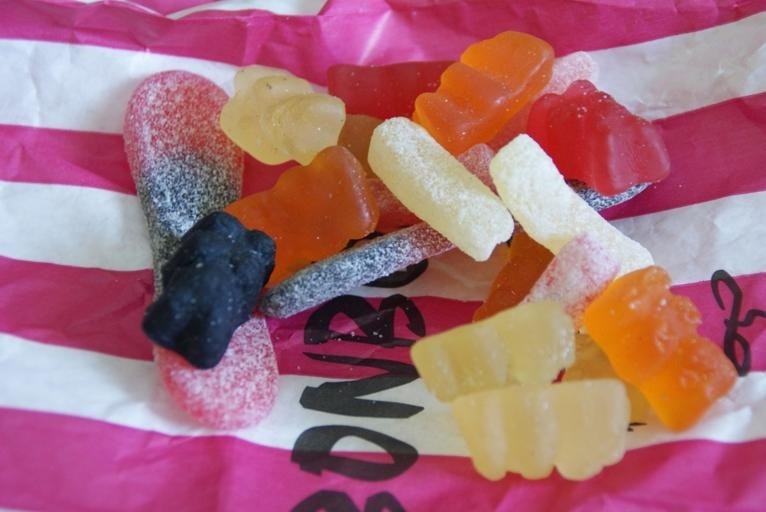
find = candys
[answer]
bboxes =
[122,28,739,480]
